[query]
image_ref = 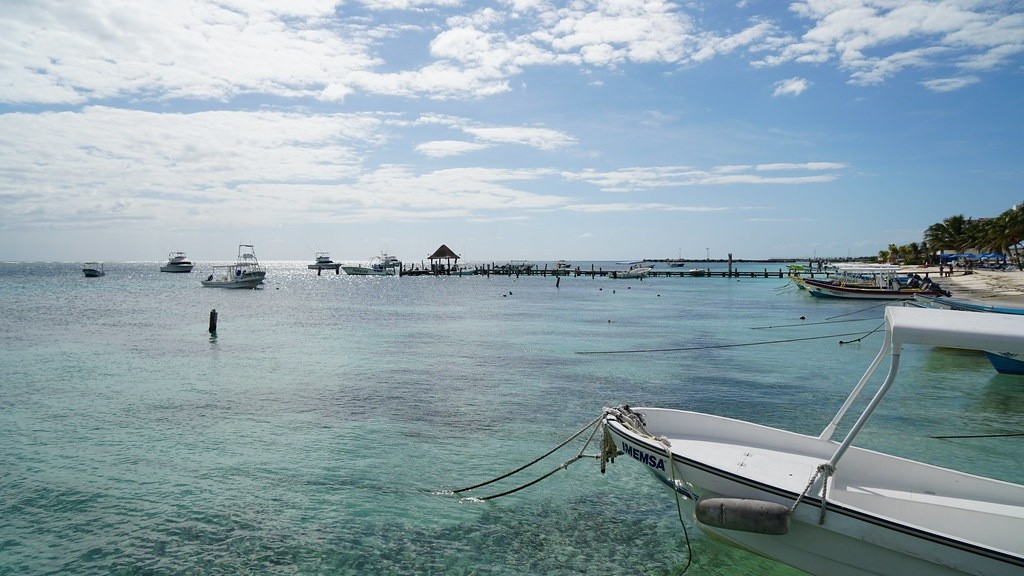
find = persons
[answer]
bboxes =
[817,259,828,273]
[906,273,922,288]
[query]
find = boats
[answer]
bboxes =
[381,252,400,267]
[669,263,685,267]
[308,252,342,269]
[600,306,1024,576]
[341,257,396,276]
[160,251,193,273]
[557,262,572,269]
[82,262,105,277]
[789,271,942,299]
[200,244,266,289]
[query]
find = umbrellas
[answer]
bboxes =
[940,252,1007,261]
